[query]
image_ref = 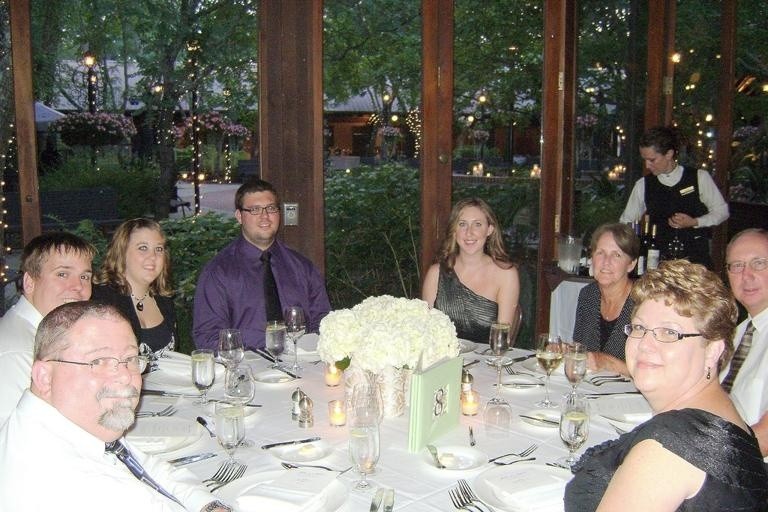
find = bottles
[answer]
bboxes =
[626,213,660,278]
[579,244,594,276]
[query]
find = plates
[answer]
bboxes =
[213,471,351,512]
[117,359,224,468]
[472,463,576,511]
[430,447,487,471]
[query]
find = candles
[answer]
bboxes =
[328,400,347,426]
[324,361,341,386]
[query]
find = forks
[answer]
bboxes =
[586,374,630,386]
[448,480,495,512]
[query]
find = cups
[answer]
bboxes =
[557,236,583,277]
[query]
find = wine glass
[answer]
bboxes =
[487,320,591,468]
[664,222,685,261]
[192,307,382,493]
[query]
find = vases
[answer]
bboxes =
[376,370,409,420]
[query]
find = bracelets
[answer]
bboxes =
[203,499,228,512]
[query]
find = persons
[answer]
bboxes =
[619,127,729,272]
[422,195,521,345]
[716,228,768,462]
[0,301,233,512]
[193,178,334,353]
[89,217,180,355]
[0,231,101,426]
[562,259,768,511]
[548,223,641,379]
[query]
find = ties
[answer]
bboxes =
[105,440,187,510]
[720,321,758,394]
[260,251,283,321]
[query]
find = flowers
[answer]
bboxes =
[316,293,463,373]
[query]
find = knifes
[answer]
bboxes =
[427,445,446,469]
[468,426,475,446]
[368,486,394,512]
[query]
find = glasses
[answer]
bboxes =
[45,355,148,374]
[624,324,702,343]
[726,259,768,273]
[241,206,280,215]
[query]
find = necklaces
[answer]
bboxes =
[129,289,155,314]
